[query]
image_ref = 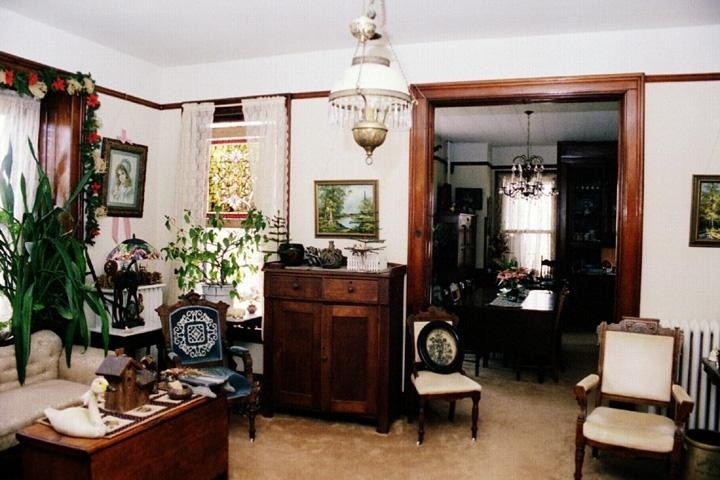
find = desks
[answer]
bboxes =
[88,324,166,366]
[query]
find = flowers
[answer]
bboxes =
[0,61,100,249]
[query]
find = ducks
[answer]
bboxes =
[43,376,117,440]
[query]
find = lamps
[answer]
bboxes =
[498,109,561,201]
[105,232,159,330]
[326,0,413,166]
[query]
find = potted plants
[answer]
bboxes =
[162,206,272,309]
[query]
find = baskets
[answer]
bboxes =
[347,255,388,273]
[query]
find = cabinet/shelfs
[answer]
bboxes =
[94,285,162,374]
[17,378,231,480]
[261,260,408,434]
[557,140,616,280]
[435,214,478,269]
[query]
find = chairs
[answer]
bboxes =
[572,317,695,478]
[405,306,479,448]
[154,288,264,444]
[444,256,577,386]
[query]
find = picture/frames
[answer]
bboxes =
[97,137,149,218]
[687,175,719,249]
[313,179,380,239]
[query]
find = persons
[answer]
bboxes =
[108,158,136,204]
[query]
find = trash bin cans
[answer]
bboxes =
[683,428,720,480]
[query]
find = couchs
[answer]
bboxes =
[0,329,128,451]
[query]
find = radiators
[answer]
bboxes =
[647,318,720,437]
